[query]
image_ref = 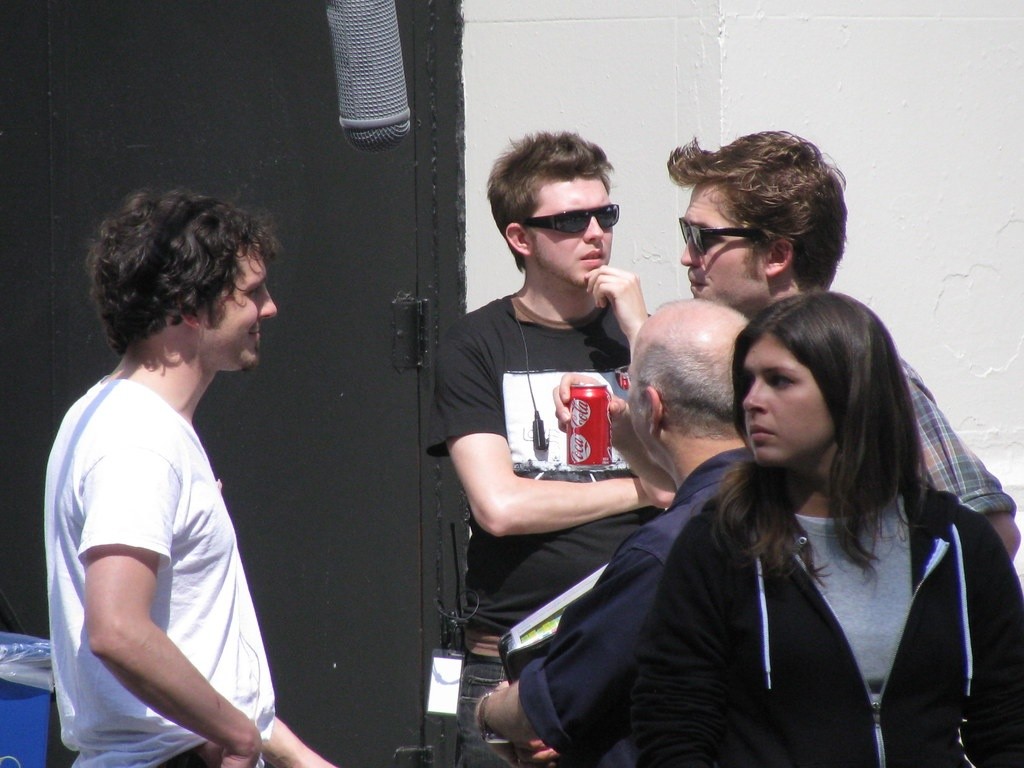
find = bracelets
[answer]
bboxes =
[479,696,497,740]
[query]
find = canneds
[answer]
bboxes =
[565,382,612,466]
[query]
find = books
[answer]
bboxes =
[497,562,609,686]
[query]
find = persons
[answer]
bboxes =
[628,292,1024,768]
[553,127,1021,564]
[475,295,757,768]
[42,185,335,768]
[423,132,667,768]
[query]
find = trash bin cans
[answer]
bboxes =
[0,631,55,768]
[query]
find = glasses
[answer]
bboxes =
[525,203,621,233]
[615,366,652,390]
[679,217,763,254]
